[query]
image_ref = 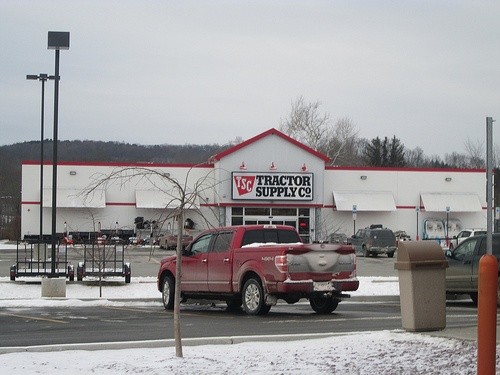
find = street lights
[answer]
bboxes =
[26,72,60,239]
[40,30,70,279]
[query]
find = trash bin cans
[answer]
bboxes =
[395,240,449,331]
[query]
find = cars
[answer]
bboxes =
[159,230,193,250]
[444,233,500,304]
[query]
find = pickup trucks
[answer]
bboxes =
[156,225,359,315]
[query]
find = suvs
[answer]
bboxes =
[346,224,397,258]
[448,229,487,249]
